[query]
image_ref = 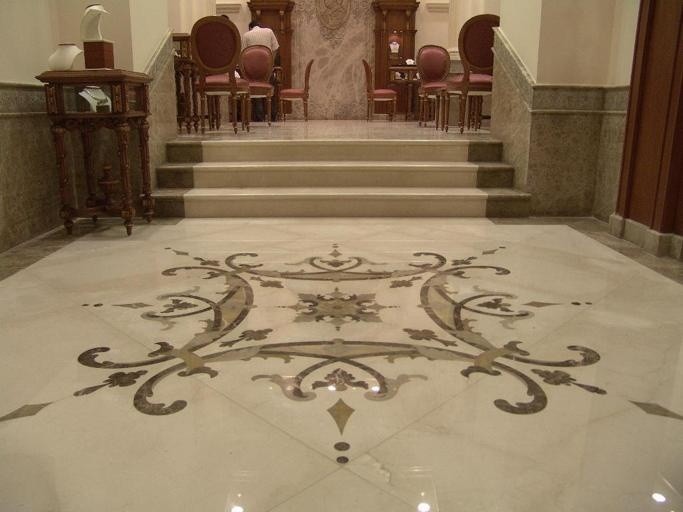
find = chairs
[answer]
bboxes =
[362,59,397,122]
[173,16,314,134]
[415,14,500,134]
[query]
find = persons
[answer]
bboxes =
[241,20,279,122]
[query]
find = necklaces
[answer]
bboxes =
[82,89,108,103]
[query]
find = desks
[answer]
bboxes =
[34,68,156,236]
[388,65,420,120]
